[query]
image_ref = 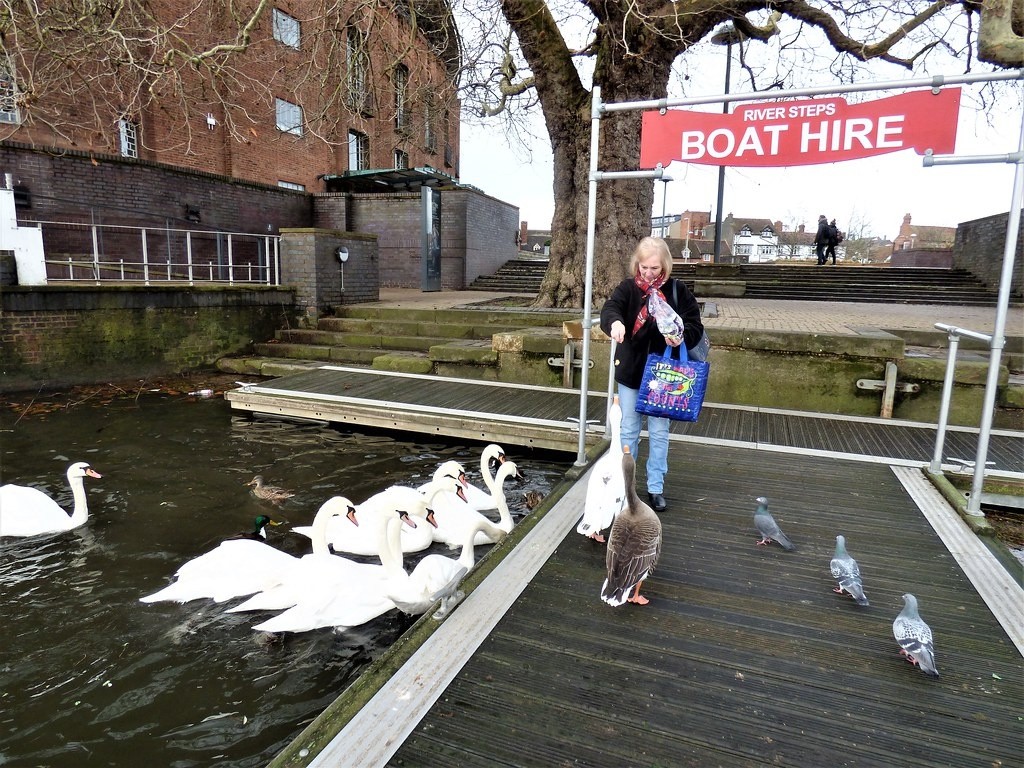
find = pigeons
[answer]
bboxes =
[829,535,869,606]
[892,594,940,677]
[753,496,797,552]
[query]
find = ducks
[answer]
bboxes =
[247,475,296,500]
[224,516,279,542]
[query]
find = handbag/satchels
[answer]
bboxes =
[634,332,709,423]
[673,278,710,360]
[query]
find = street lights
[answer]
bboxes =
[909,233,917,249]
[658,175,675,238]
[709,19,749,264]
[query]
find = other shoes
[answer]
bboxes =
[817,259,828,265]
[830,263,835,265]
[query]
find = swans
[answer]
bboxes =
[0,461,104,537]
[252,443,525,632]
[600,445,663,607]
[139,495,360,606]
[576,394,627,543]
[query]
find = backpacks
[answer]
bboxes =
[835,228,843,243]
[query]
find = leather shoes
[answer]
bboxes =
[648,492,667,512]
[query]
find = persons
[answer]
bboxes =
[822,218,840,266]
[814,215,828,266]
[597,234,706,516]
